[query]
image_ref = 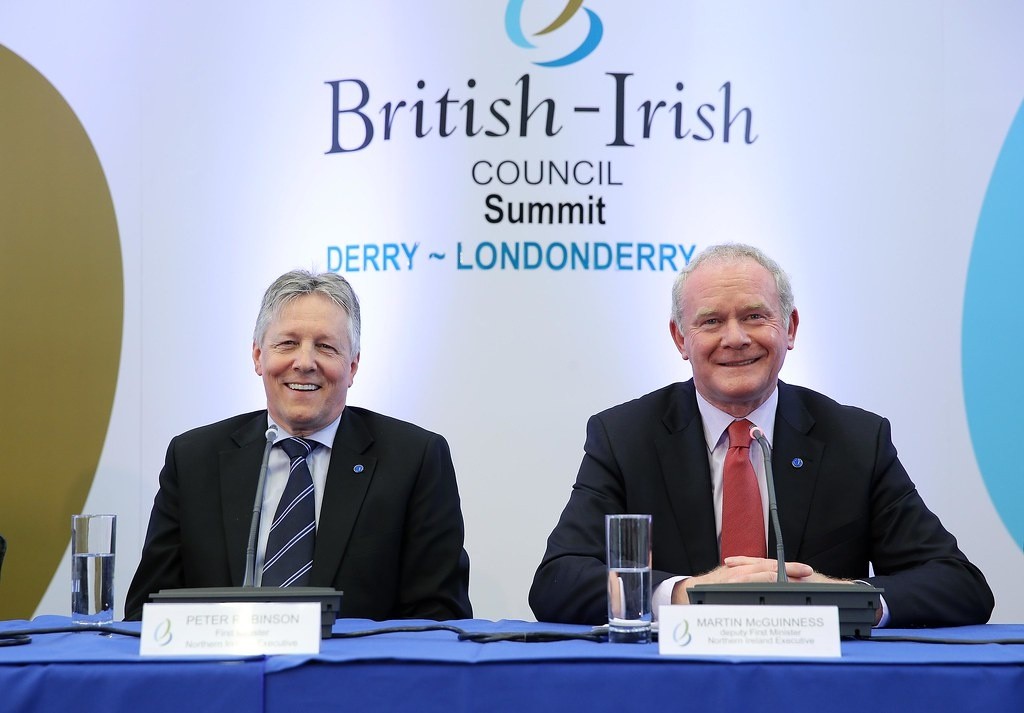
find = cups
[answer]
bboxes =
[71,514,117,624]
[605,513,653,645]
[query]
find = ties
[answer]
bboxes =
[259,437,319,588]
[720,419,767,565]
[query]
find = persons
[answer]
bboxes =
[528,244,995,632]
[125,269,473,621]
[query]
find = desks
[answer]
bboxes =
[0,618,1024,713]
